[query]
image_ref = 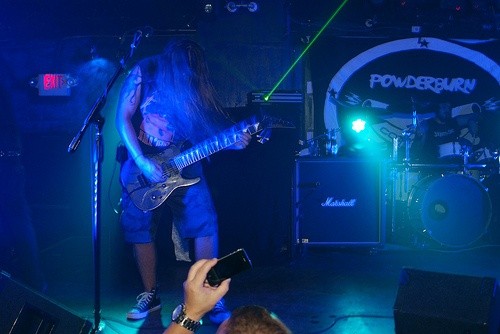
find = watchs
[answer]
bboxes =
[170,304,203,332]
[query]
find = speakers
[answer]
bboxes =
[209,107,302,257]
[393,267,496,334]
[293,156,385,252]
[0,270,95,334]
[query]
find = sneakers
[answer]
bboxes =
[206,296,229,323]
[127,287,161,319]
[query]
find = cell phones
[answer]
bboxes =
[205,248,253,287]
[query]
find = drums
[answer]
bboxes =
[407,172,492,248]
[471,145,500,164]
[438,142,473,163]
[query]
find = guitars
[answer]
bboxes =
[120,113,275,213]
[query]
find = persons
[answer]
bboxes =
[161,258,293,334]
[116,38,252,324]
[411,96,479,168]
